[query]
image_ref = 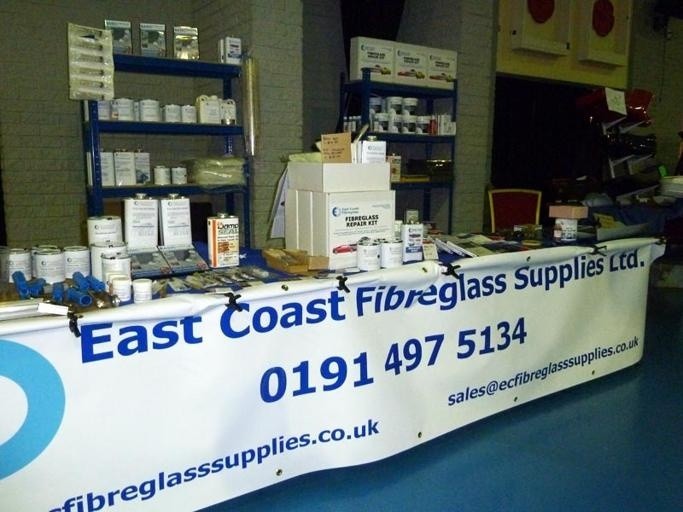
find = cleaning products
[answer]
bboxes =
[124,192,158,248]
[87,145,151,186]
[195,95,237,124]
[158,193,193,247]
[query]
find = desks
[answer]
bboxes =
[1,228,667,510]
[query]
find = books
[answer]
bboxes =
[130,246,273,299]
[427,228,532,258]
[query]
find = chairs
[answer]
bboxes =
[486,187,542,234]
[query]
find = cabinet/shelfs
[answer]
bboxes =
[79,36,250,251]
[338,67,457,236]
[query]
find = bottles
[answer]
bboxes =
[343,115,361,131]
[369,95,430,135]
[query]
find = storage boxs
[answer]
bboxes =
[283,161,396,270]
